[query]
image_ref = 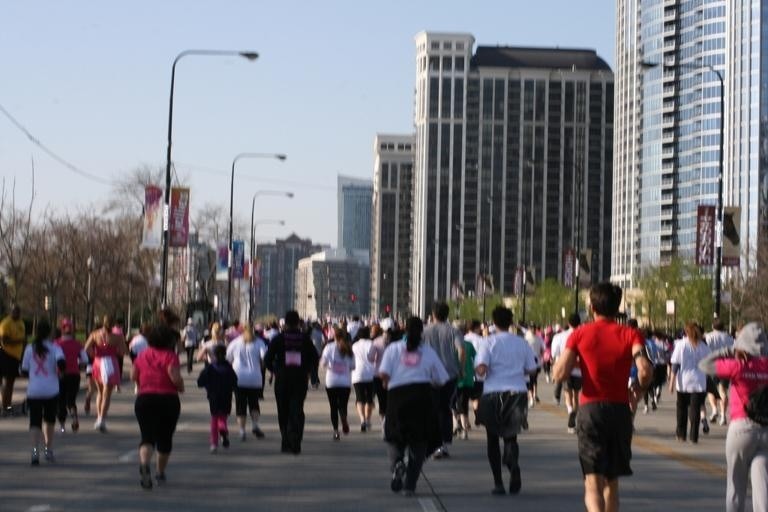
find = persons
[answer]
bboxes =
[0,304,27,413]
[53,318,89,433]
[21,320,66,465]
[84,283,734,512]
[697,321,768,512]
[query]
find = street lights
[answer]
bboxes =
[226,152,290,319]
[634,56,730,325]
[86,252,95,338]
[156,47,260,306]
[253,217,287,268]
[246,185,298,318]
[362,157,584,330]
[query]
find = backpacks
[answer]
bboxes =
[731,358,768,427]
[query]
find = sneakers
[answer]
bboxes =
[568,410,577,434]
[530,396,539,408]
[209,426,263,454]
[334,422,371,440]
[391,460,415,496]
[138,462,166,489]
[431,420,470,457]
[30,411,106,465]
[675,414,726,444]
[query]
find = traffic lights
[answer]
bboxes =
[351,293,355,304]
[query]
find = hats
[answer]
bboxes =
[733,322,767,357]
[60,318,72,333]
